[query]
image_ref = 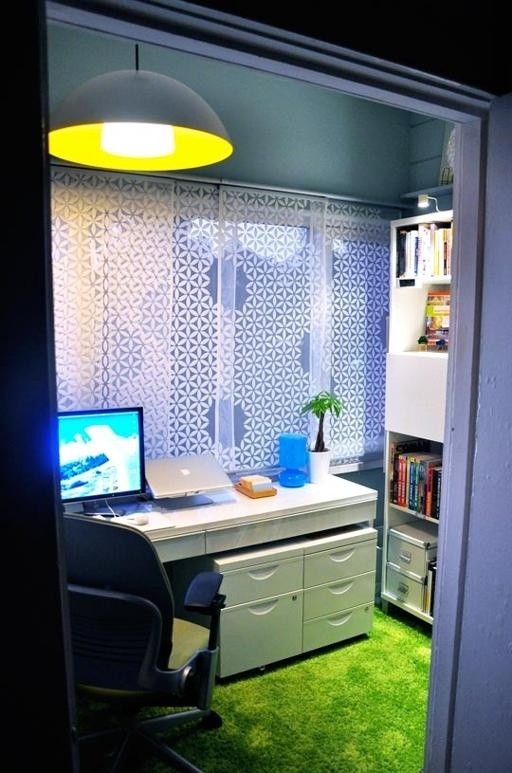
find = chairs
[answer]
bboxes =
[64,511,226,773]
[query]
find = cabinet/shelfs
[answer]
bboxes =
[381,210,456,626]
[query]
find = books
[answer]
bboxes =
[413,290,454,349]
[396,220,458,277]
[388,437,444,522]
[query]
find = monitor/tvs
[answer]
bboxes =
[57,406,147,517]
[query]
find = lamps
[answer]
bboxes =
[49,43,233,173]
[418,194,440,212]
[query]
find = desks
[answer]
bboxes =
[93,473,378,682]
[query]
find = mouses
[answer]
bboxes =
[125,512,149,525]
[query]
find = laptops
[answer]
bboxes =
[144,452,234,500]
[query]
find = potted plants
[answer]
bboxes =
[300,391,342,474]
